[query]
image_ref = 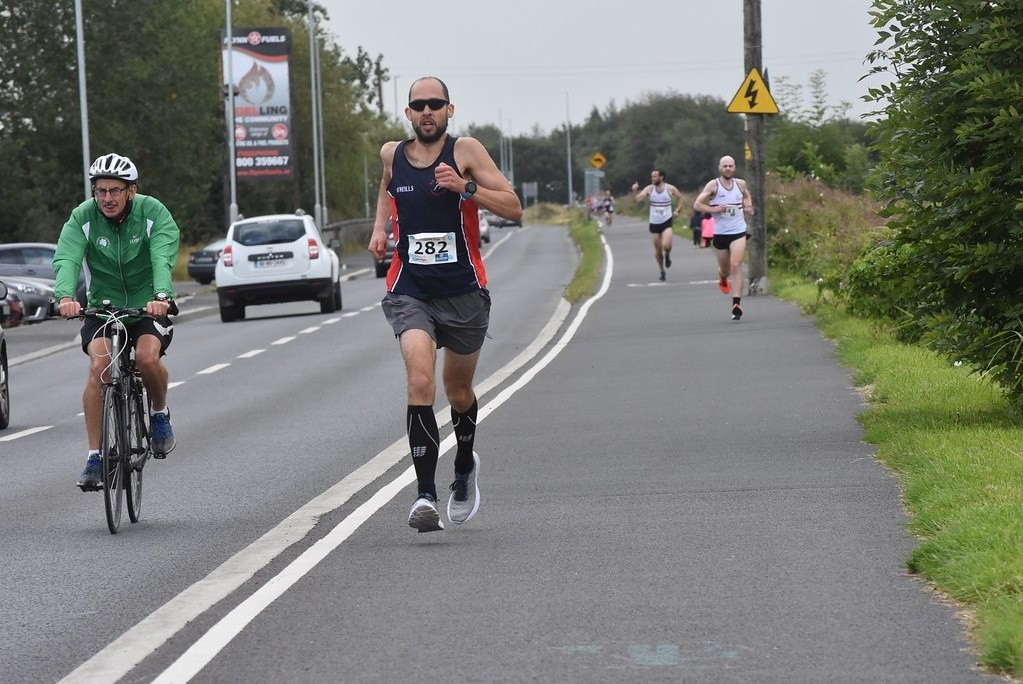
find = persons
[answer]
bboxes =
[586,190,616,228]
[53,153,181,486]
[690,155,754,320]
[368,77,522,533]
[631,168,685,282]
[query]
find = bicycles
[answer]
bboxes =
[49,298,182,535]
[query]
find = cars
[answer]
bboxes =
[477,207,522,242]
[187,240,229,286]
[0,281,21,431]
[1,274,60,332]
[369,217,396,279]
[1,243,89,314]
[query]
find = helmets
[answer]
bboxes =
[88,153,138,184]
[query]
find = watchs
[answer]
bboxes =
[461,179,477,198]
[155,292,170,302]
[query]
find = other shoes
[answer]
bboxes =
[730,304,743,323]
[665,255,671,267]
[658,271,666,280]
[719,274,730,294]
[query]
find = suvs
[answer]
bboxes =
[215,209,345,321]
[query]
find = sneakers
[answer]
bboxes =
[76,452,104,486]
[447,451,481,524]
[406,492,445,533]
[148,407,176,456]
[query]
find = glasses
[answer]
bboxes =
[408,98,448,112]
[93,185,127,197]
